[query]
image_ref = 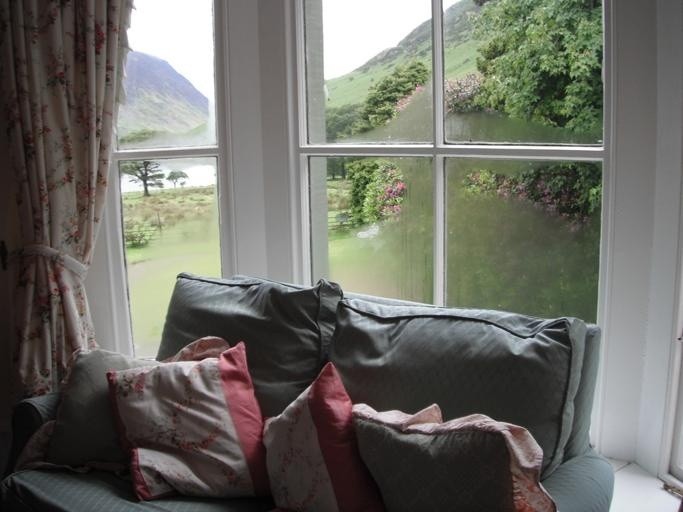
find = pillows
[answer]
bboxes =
[351,402,556,512]
[153,272,342,421]
[322,299,587,481]
[106,340,274,502]
[15,345,167,478]
[261,361,386,512]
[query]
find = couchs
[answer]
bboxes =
[0,270,615,512]
